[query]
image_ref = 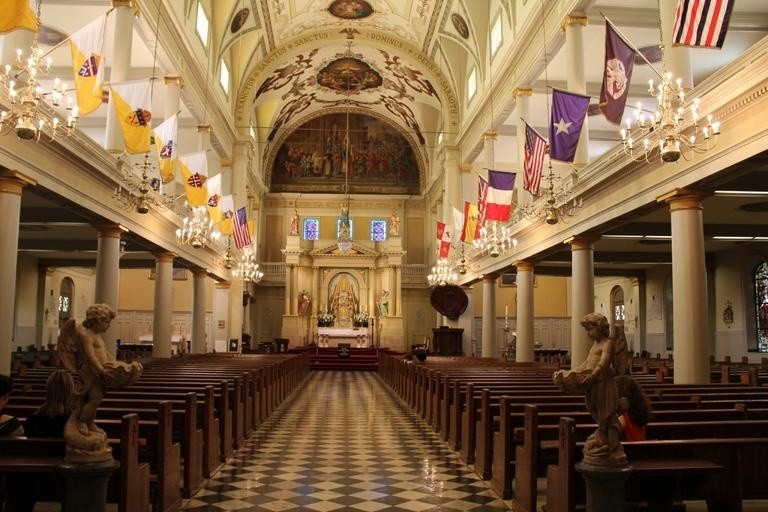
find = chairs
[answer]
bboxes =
[411,337,431,355]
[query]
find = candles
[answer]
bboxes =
[505,304,508,326]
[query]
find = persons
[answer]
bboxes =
[72,302,127,436]
[566,311,618,454]
[275,142,413,187]
[402,343,429,367]
[177,336,188,355]
[21,370,79,438]
[611,374,650,442]
[1,373,27,438]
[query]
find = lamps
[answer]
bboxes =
[424,0,461,288]
[449,0,482,276]
[110,1,177,216]
[472,0,518,260]
[511,0,582,225]
[334,39,354,253]
[0,1,80,145]
[217,1,264,284]
[175,2,223,248]
[618,1,720,165]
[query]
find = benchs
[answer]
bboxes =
[377,344,768,511]
[1,339,310,511]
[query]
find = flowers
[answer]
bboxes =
[317,313,331,324]
[352,311,369,322]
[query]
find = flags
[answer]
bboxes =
[0,0,43,38]
[66,13,104,115]
[545,88,592,164]
[461,202,483,247]
[111,77,154,155]
[215,194,235,236]
[232,207,252,249]
[476,177,495,215]
[434,222,454,259]
[522,123,548,198]
[598,21,637,127]
[152,113,179,184]
[176,150,210,208]
[484,170,517,224]
[670,0,737,52]
[452,207,466,240]
[204,173,221,221]
